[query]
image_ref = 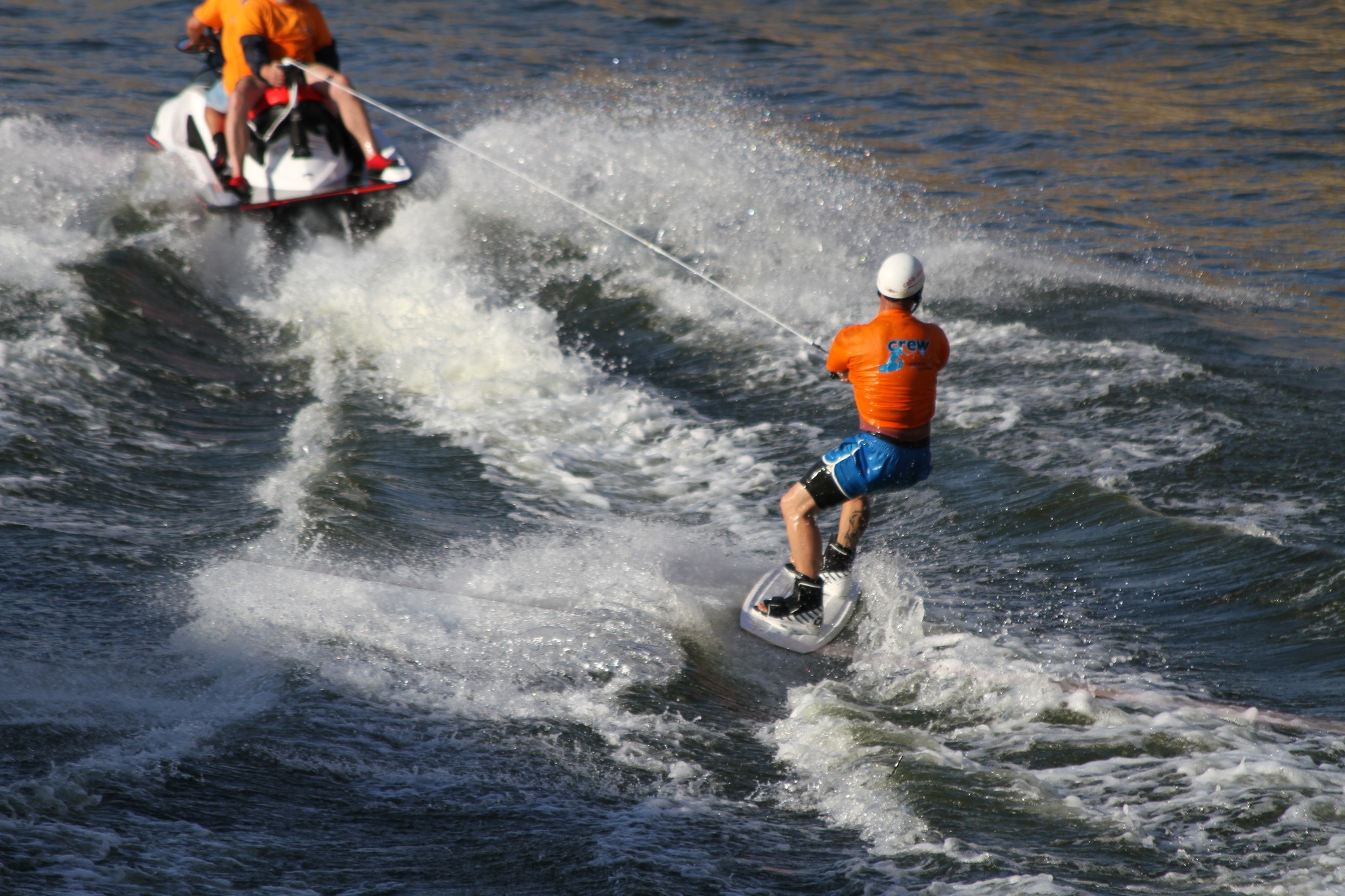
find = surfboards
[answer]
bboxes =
[740,554,862,654]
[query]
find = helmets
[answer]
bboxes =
[876,252,925,300]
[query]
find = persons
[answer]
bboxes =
[754,252,951,626]
[186,0,412,199]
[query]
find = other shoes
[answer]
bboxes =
[754,563,825,635]
[783,542,853,597]
[212,150,228,168]
[225,176,252,196]
[366,154,397,173]
